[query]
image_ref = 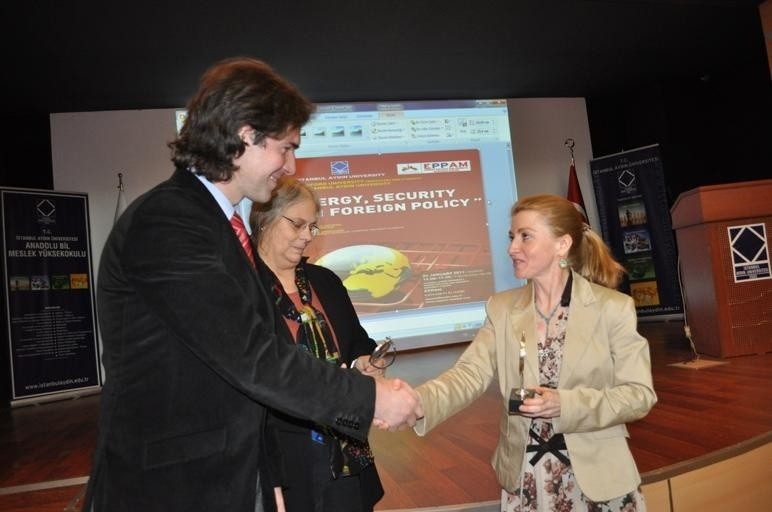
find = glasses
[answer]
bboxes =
[282,214,322,238]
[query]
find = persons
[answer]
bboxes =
[373,194,659,512]
[82,59,424,512]
[249,175,385,512]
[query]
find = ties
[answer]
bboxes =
[230,212,258,279]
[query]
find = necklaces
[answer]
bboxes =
[534,301,565,338]
